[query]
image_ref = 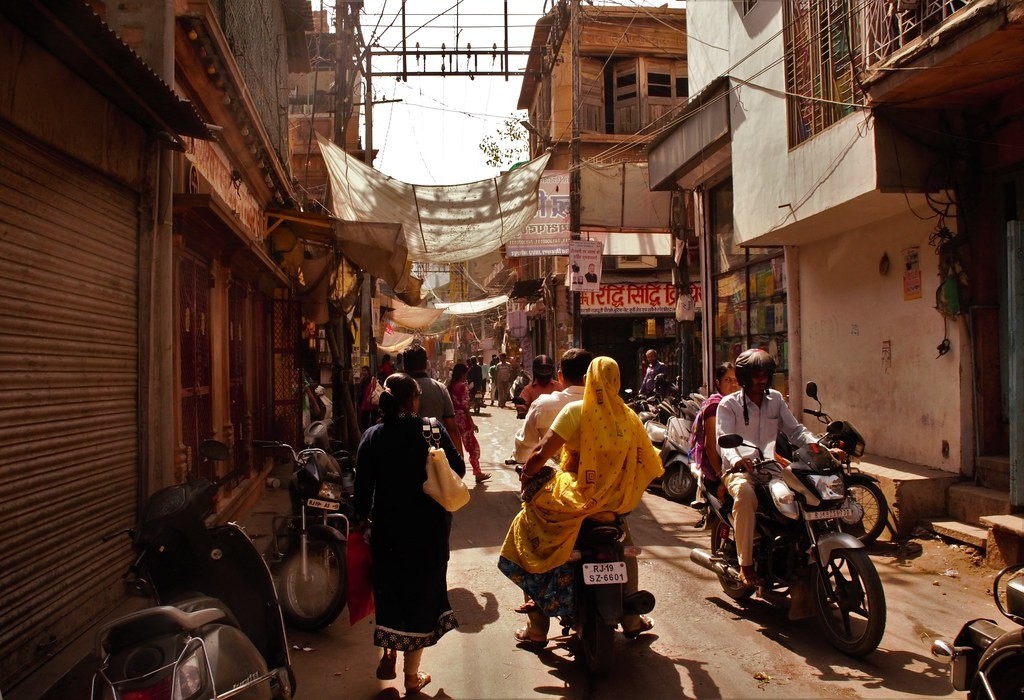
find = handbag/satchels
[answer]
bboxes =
[345,531,376,628]
[421,416,471,512]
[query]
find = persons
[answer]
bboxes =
[509,347,655,647]
[447,364,492,482]
[572,260,583,284]
[496,356,665,615]
[489,354,512,408]
[394,353,404,373]
[701,362,741,485]
[402,346,455,435]
[517,355,564,418]
[380,354,393,375]
[715,348,848,586]
[301,367,319,428]
[636,349,671,397]
[351,373,466,696]
[458,355,491,403]
[359,366,379,428]
[585,263,597,283]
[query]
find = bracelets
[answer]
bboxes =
[522,464,533,477]
[716,471,722,476]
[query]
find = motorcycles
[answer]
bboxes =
[622,372,1024,700]
[504,458,657,680]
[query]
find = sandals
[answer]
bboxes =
[516,601,540,612]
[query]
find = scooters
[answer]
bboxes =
[467,382,487,416]
[251,414,360,633]
[87,439,297,700]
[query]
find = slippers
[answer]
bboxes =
[376,655,396,680]
[406,673,430,693]
[513,626,549,648]
[624,617,654,639]
[738,574,762,586]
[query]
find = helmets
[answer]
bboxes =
[533,354,553,383]
[735,348,775,388]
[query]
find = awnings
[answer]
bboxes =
[510,278,545,297]
[268,208,421,304]
